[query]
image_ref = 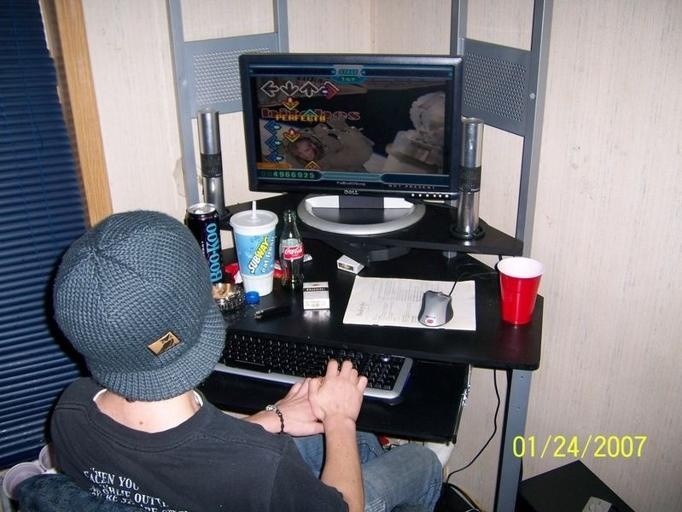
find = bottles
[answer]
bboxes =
[279,209,304,290]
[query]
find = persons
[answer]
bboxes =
[287,131,327,167]
[45,207,446,512]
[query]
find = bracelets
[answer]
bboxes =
[261,402,286,437]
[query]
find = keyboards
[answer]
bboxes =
[216,322,415,405]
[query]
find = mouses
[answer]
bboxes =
[415,288,454,327]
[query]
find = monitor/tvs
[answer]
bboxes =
[236,52,464,239]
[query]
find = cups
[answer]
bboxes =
[230,209,279,298]
[497,256,544,326]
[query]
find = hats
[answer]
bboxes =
[51,210,227,402]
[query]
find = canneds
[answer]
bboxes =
[184,203,224,287]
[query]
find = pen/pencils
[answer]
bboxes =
[255,302,293,318]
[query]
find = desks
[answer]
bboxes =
[183,193,546,510]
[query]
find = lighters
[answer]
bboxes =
[254,301,289,320]
